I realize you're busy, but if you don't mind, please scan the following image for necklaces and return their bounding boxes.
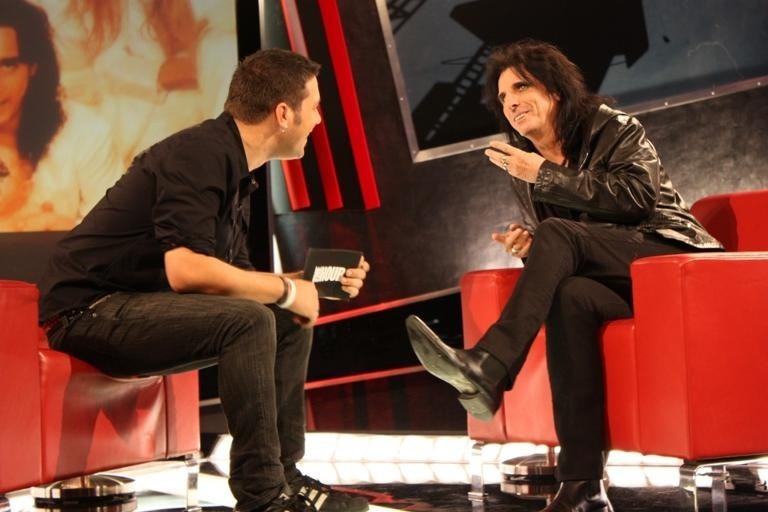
[559,153,568,167]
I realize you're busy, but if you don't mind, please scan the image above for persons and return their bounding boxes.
[0,0,115,234]
[404,36,728,511]
[33,45,372,512]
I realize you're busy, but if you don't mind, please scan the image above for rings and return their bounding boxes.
[511,246,520,254]
[500,163,508,172]
[501,155,509,164]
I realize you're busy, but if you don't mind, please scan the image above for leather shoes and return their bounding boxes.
[540,480,616,512]
[404,313,509,423]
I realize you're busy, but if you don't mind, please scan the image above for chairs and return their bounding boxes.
[1,278,202,510]
[458,188,767,504]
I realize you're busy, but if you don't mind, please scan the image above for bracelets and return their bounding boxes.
[297,269,304,280]
[274,276,297,310]
[276,275,289,306]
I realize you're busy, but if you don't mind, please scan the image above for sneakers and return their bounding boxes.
[291,474,369,512]
[232,486,316,512]
